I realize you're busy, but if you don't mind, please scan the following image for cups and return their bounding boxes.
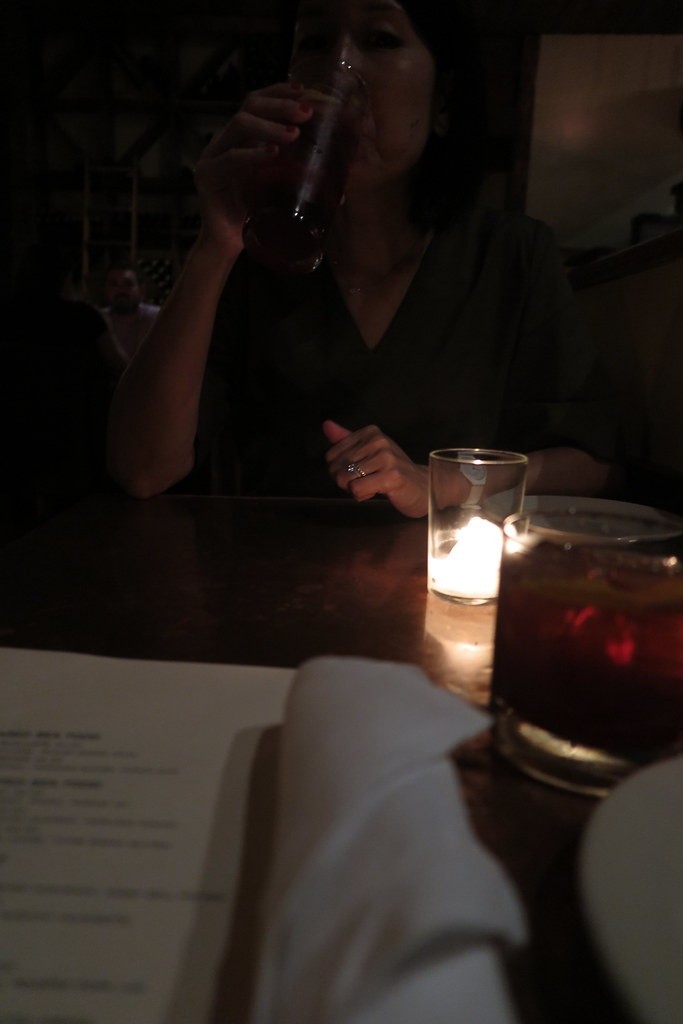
[426,447,528,603]
[243,55,373,271]
[485,507,683,798]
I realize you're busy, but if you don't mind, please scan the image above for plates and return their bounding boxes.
[523,494,683,549]
[576,756,683,1023]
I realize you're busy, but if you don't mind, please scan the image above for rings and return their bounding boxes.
[345,463,366,477]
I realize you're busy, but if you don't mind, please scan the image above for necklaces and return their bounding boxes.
[347,267,394,294]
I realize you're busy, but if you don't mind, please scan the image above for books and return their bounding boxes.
[0,647,531,1023]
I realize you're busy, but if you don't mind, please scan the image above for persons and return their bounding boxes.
[0,243,162,549]
[106,1,649,520]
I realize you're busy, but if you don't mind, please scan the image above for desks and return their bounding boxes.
[0,493,426,668]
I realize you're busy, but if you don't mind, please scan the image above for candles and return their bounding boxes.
[426,448,529,605]
